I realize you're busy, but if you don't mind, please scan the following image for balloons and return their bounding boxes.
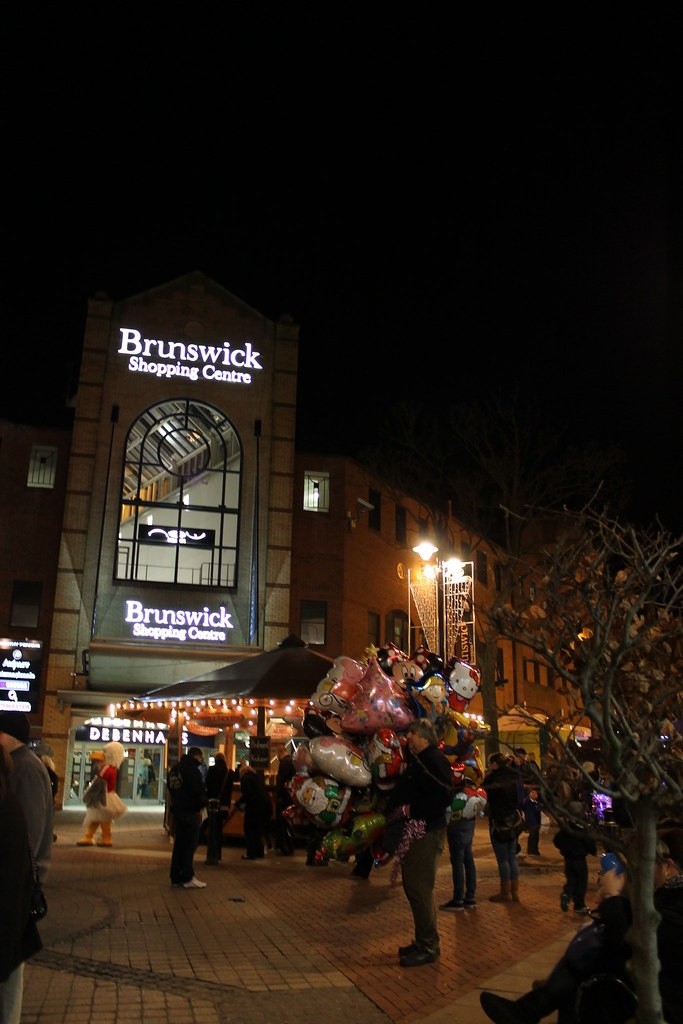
[280,640,489,846]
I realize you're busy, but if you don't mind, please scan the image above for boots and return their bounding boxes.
[489,883,512,902]
[512,882,520,901]
[480,992,538,1024]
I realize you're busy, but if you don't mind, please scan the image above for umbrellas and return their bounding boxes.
[130,629,335,703]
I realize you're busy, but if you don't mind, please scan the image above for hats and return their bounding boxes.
[0,710,29,744]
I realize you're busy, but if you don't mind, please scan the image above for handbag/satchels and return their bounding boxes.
[208,799,220,812]
[29,883,49,922]
[505,809,526,833]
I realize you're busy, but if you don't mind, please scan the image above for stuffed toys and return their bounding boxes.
[74,741,129,847]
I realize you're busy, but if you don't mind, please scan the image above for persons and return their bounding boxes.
[166,748,207,889]
[479,850,683,1023]
[0,710,59,1024]
[235,765,274,859]
[440,764,478,909]
[390,721,453,964]
[552,802,603,915]
[137,757,157,799]
[268,746,295,856]
[204,753,241,864]
[304,822,375,881]
[483,745,543,903]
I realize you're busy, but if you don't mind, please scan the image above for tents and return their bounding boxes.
[479,703,573,769]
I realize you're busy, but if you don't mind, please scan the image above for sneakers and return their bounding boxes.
[439,900,464,911]
[463,899,477,908]
[399,943,440,967]
[171,877,207,888]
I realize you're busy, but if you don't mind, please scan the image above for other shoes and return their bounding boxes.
[242,854,250,859]
[561,893,568,911]
[528,851,540,855]
[575,907,587,912]
[204,856,221,865]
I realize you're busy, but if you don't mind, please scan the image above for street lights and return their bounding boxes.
[412,514,466,667]
